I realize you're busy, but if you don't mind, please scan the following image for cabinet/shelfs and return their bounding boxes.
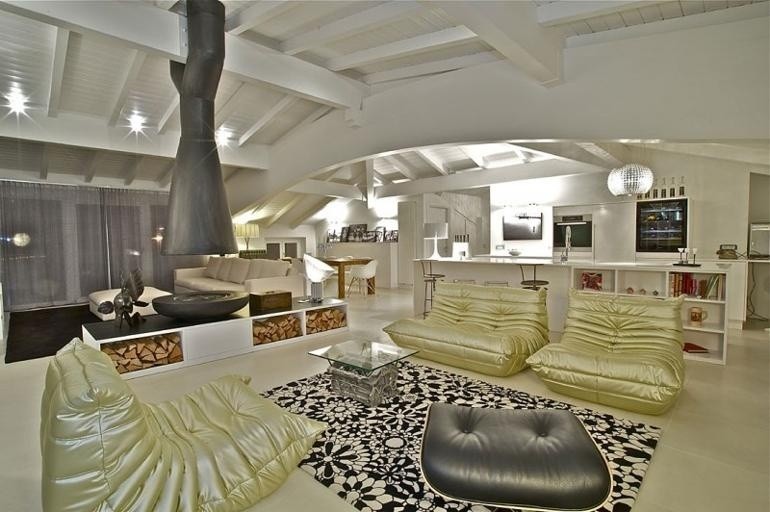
[567,267,729,367]
[82,301,350,380]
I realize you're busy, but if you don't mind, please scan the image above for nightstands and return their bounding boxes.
[308,339,420,408]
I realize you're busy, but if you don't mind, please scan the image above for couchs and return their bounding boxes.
[171,263,303,297]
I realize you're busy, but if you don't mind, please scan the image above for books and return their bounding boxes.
[682,342,709,353]
[670,272,725,300]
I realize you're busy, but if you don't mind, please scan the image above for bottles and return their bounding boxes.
[637,177,685,199]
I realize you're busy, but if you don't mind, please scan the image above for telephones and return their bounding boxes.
[717,249,737,259]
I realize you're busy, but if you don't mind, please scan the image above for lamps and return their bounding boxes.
[603,164,656,199]
[302,252,334,303]
[233,221,260,249]
[423,220,450,260]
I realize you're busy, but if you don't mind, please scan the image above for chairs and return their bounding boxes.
[319,253,379,297]
[518,263,551,306]
[419,257,443,318]
[37,330,325,510]
[532,288,686,415]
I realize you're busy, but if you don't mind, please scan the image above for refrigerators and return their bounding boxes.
[634,200,687,257]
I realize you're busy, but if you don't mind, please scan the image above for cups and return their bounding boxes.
[690,306,709,328]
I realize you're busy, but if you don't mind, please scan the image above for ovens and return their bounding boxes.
[553,215,594,253]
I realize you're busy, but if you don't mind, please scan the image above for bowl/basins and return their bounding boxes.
[152,289,250,319]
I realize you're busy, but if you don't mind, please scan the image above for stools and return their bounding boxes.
[418,403,613,511]
[88,286,173,321]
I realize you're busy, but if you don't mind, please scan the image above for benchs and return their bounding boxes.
[381,278,549,378]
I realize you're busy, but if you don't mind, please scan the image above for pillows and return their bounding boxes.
[203,256,286,282]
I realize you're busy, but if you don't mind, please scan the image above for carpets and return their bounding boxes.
[259,362,665,512]
[2,303,102,364]
